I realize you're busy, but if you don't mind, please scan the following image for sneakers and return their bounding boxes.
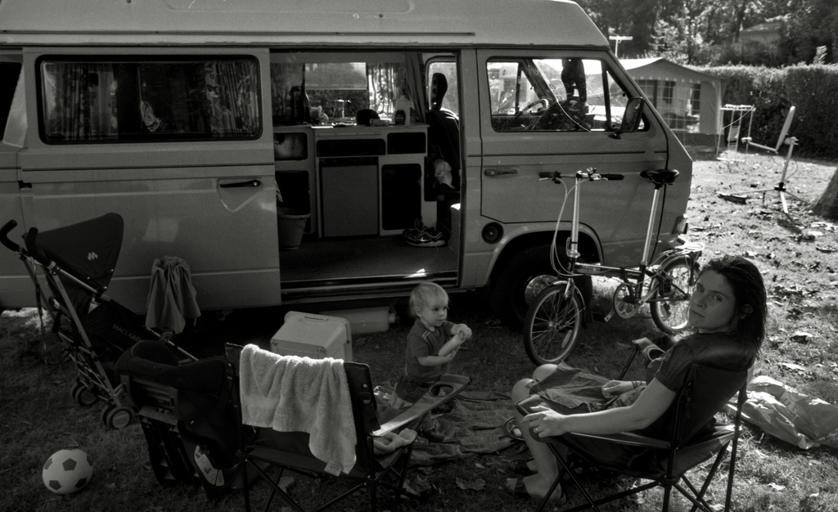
[421,427,444,441]
[406,226,446,247]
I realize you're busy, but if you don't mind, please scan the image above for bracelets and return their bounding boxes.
[632,380,643,389]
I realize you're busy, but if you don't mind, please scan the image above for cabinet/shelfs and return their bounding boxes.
[273,126,321,240]
[377,123,432,235]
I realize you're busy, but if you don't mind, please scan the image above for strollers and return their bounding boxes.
[1,204,197,431]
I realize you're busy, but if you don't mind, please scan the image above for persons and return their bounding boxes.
[283,86,329,124]
[503,254,768,506]
[396,282,477,415]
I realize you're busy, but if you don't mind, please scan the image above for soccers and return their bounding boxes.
[41,450,94,495]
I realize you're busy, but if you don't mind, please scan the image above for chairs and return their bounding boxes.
[428,74,463,185]
[518,318,745,512]
[228,344,472,512]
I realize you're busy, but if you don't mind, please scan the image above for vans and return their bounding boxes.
[1,5,691,336]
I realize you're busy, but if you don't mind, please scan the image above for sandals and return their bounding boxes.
[510,459,538,475]
[504,477,566,505]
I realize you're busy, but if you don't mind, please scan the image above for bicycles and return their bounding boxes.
[520,160,715,370]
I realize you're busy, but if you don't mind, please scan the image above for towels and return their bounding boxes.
[236,347,363,470]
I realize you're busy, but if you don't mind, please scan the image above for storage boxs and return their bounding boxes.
[270,309,353,363]
